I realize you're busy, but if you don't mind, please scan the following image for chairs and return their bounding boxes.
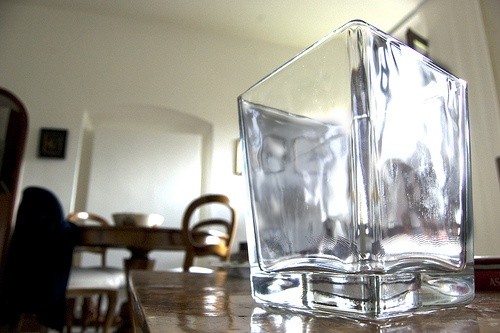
[183,193,237,270]
[68,212,109,332]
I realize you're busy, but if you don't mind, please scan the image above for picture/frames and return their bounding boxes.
[38,128,67,158]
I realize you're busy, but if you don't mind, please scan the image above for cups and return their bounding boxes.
[236,19,475,322]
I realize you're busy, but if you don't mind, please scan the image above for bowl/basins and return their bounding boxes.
[111,212,165,228]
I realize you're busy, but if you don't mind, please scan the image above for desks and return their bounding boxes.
[128,269,500,333]
[71,226,186,333]
